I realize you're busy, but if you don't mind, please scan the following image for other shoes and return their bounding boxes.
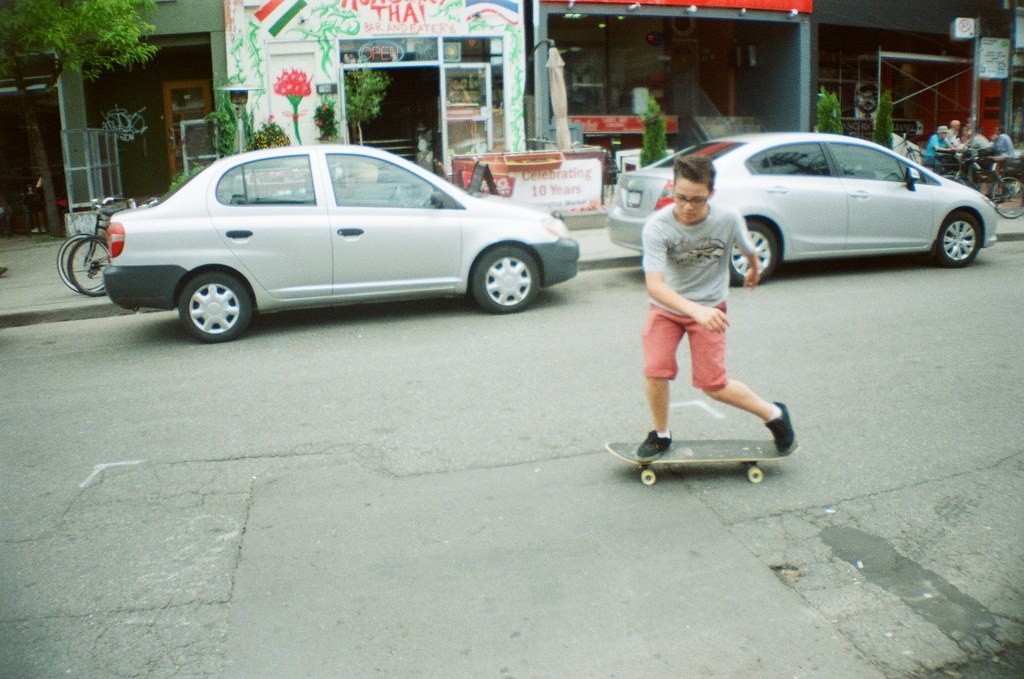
[0,265,7,276]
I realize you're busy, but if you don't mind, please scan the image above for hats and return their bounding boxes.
[937,126,950,133]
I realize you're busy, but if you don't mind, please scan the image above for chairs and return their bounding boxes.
[0,186,49,238]
[934,150,1024,183]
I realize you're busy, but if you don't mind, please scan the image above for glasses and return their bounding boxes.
[673,194,710,208]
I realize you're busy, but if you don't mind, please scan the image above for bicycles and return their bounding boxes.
[56,197,155,297]
[941,151,1024,219]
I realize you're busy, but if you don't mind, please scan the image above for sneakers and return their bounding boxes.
[636,430,672,462]
[766,402,796,456]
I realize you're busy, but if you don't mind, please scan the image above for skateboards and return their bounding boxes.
[604,438,801,487]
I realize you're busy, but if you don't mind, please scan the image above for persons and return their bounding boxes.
[635,156,796,463]
[922,120,1014,174]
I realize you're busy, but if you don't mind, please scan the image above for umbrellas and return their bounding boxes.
[546,39,572,150]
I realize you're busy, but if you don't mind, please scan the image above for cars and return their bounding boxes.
[604,131,997,286]
[101,143,581,345]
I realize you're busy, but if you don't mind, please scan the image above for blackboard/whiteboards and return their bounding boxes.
[468,161,498,195]
